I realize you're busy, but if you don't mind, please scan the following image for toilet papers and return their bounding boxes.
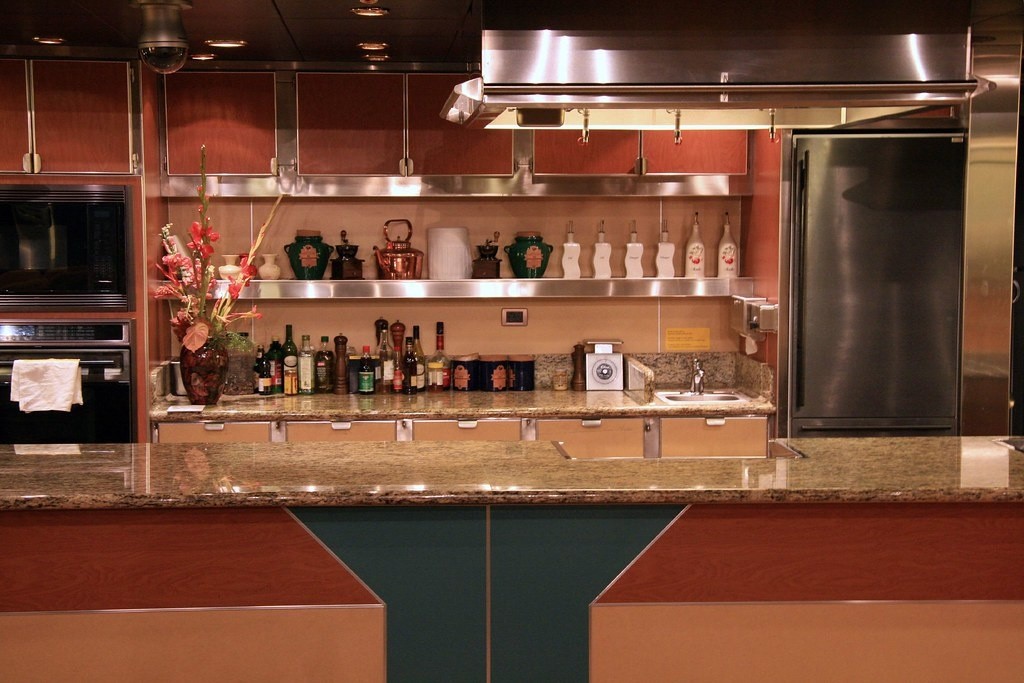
[427,226,475,280]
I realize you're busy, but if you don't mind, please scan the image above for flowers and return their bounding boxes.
[152,144,283,354]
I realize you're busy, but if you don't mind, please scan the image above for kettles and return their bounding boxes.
[373,218,423,280]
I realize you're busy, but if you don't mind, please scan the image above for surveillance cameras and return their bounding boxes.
[137,3,190,74]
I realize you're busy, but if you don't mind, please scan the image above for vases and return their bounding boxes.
[180,339,228,405]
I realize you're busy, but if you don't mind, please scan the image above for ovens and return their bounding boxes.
[1,318,133,445]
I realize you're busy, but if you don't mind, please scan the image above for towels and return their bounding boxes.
[10,358,84,414]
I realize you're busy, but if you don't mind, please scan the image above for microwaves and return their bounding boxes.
[1,183,134,312]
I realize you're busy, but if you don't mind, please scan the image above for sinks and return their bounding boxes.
[664,395,740,402]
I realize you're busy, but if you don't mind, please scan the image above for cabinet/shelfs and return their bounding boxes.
[0,57,142,183]
[155,60,754,199]
[159,415,770,458]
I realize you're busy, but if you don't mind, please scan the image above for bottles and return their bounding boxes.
[504,231,553,278]
[684,213,705,278]
[258,253,281,280]
[552,368,568,391]
[717,215,738,278]
[624,220,644,278]
[253,322,451,395]
[238,253,257,279]
[218,254,240,280]
[591,220,612,279]
[655,220,675,278]
[561,220,581,279]
[284,230,334,280]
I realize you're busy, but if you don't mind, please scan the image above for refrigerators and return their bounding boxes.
[782,132,970,438]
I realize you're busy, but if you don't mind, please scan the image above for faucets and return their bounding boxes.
[690,358,706,392]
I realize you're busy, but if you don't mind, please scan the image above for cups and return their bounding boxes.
[451,360,535,391]
